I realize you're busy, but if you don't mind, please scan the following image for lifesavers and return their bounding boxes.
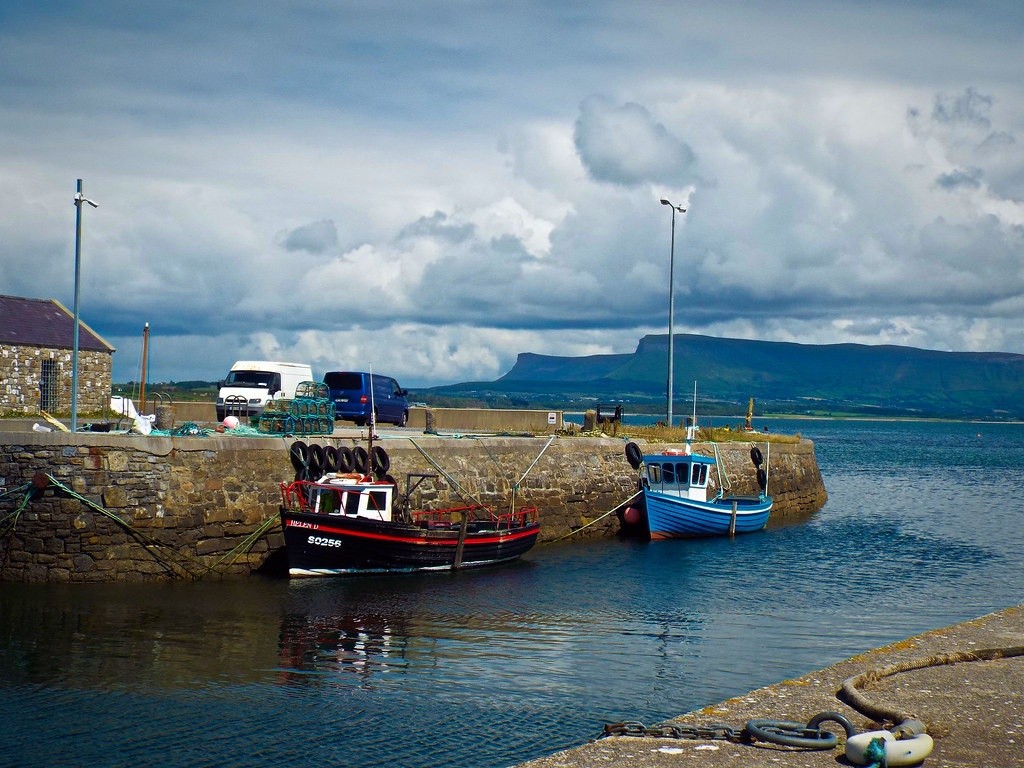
[750,447,764,466]
[625,441,644,466]
[757,468,767,490]
[289,439,399,506]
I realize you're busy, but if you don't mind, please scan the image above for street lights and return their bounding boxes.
[70,178,101,433]
[659,196,687,427]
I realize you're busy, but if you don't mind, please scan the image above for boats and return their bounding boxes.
[635,379,774,542]
[277,362,543,578]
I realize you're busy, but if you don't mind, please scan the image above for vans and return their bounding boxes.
[322,371,410,427]
[214,360,314,422]
